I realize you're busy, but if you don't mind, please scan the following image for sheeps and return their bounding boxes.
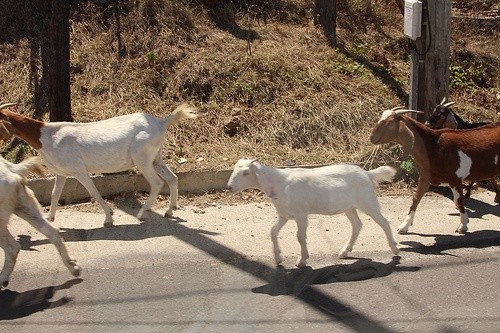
[370,106,500,234]
[0,102,201,227]
[0,154,83,288]
[424,97,500,206]
[227,158,400,268]
